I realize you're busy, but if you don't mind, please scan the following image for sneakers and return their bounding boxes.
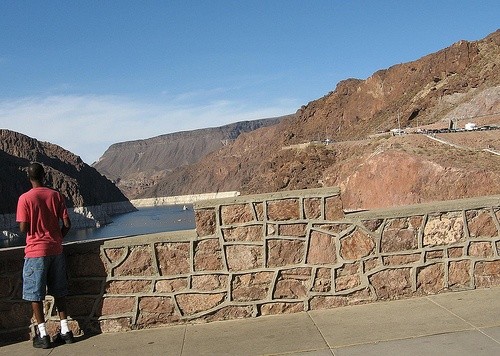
[33,331,51,349]
[52,329,75,344]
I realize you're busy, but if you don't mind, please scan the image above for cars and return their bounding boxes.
[427,123,495,134]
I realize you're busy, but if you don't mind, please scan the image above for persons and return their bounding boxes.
[16,162,75,349]
